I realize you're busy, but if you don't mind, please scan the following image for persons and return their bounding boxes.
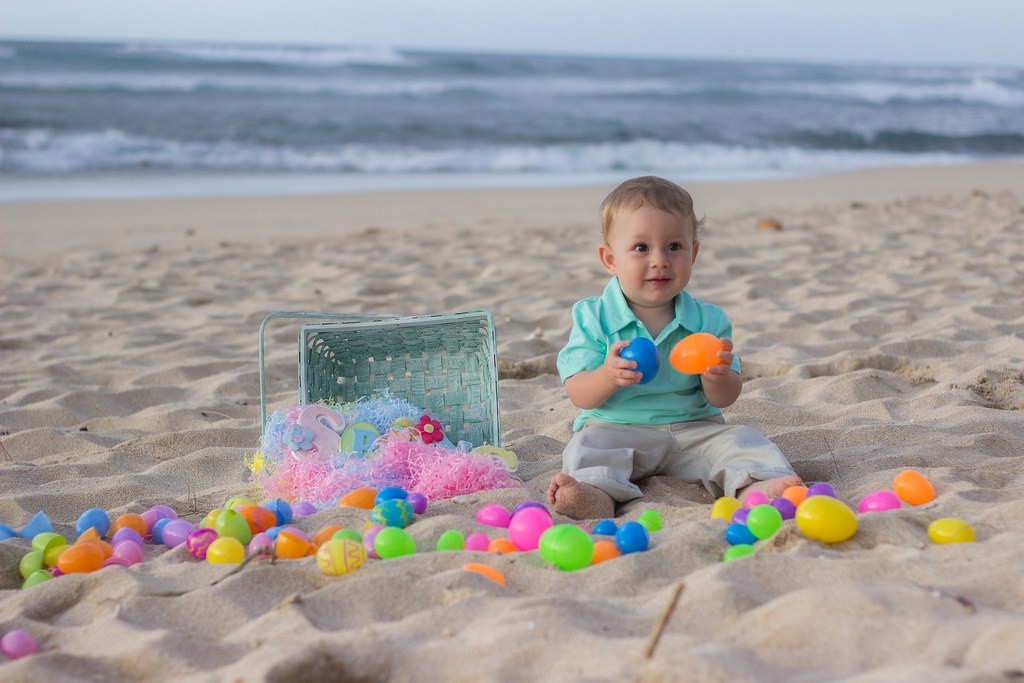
[547,175,806,521]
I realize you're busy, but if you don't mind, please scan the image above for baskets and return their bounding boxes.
[258,311,500,454]
